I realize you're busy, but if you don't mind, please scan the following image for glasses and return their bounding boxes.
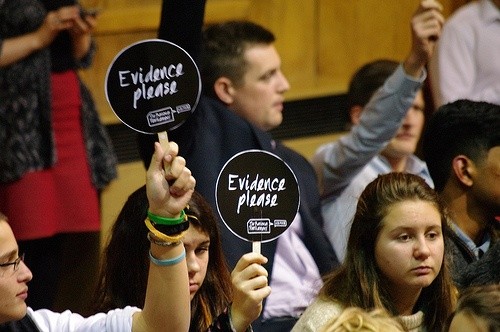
[0,253,24,271]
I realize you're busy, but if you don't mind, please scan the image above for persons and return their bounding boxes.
[291,0,500,332]
[90,180,271,332]
[0,0,117,318]
[135,1,341,332]
[0,141,196,332]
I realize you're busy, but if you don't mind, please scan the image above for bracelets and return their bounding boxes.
[145,208,189,266]
